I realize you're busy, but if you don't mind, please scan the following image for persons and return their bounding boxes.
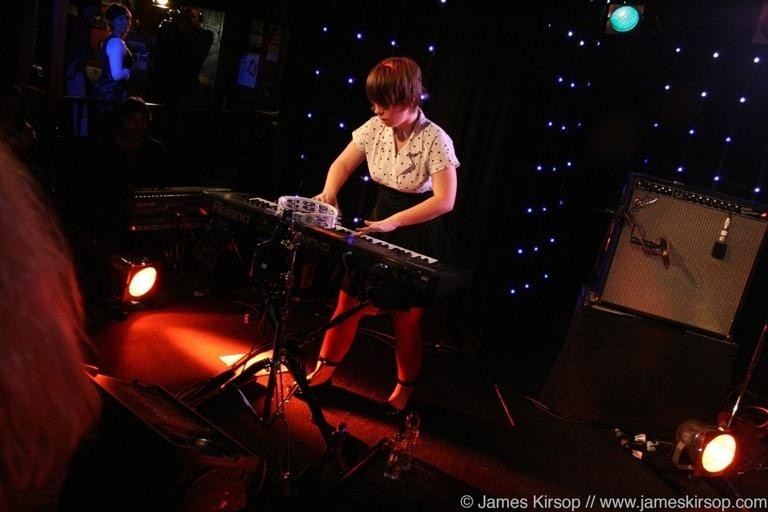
[0,85,36,158]
[287,56,461,421]
[63,0,214,104]
[0,141,103,512]
[104,96,167,186]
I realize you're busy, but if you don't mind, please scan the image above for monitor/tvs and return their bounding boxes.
[235,47,262,91]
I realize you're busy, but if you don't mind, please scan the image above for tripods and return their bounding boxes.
[194,251,337,449]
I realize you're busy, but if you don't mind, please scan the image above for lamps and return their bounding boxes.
[669,416,738,477]
[99,249,160,305]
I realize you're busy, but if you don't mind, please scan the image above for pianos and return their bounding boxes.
[212,192,450,295]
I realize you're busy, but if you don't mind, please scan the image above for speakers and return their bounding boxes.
[597,168,768,340]
[80,370,260,512]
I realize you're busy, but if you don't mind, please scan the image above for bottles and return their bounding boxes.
[615,428,632,456]
[384,409,420,481]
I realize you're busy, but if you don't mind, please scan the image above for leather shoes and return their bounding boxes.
[376,388,415,418]
[287,376,333,396]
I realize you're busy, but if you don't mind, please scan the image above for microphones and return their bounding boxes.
[710,212,734,258]
[630,235,670,258]
